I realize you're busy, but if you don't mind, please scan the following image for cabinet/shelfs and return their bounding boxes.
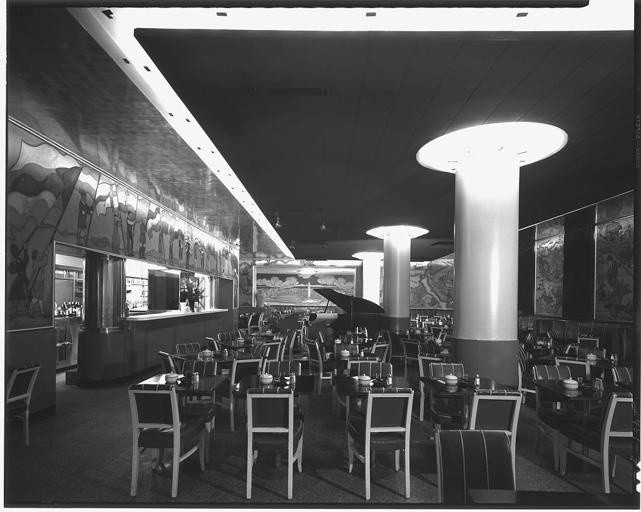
[54,317,82,370]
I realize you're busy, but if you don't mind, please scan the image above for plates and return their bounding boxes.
[284,376,290,380]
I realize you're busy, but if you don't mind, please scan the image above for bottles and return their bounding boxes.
[250,338,253,345]
[224,349,228,357]
[385,376,389,386]
[290,373,296,384]
[57,305,63,316]
[55,300,58,316]
[474,374,481,387]
[405,329,409,334]
[191,373,197,383]
[195,372,200,382]
[595,378,599,390]
[598,379,603,391]
[198,352,203,360]
[274,333,277,340]
[63,300,81,317]
[387,374,392,385]
[278,332,281,339]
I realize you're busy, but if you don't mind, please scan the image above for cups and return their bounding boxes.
[360,349,365,358]
[256,368,263,377]
[487,379,495,392]
[350,337,354,345]
[352,377,359,385]
[211,351,215,358]
[193,307,201,312]
[194,302,198,307]
[577,376,583,382]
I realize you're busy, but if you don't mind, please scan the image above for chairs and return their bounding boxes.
[5,363,41,447]
